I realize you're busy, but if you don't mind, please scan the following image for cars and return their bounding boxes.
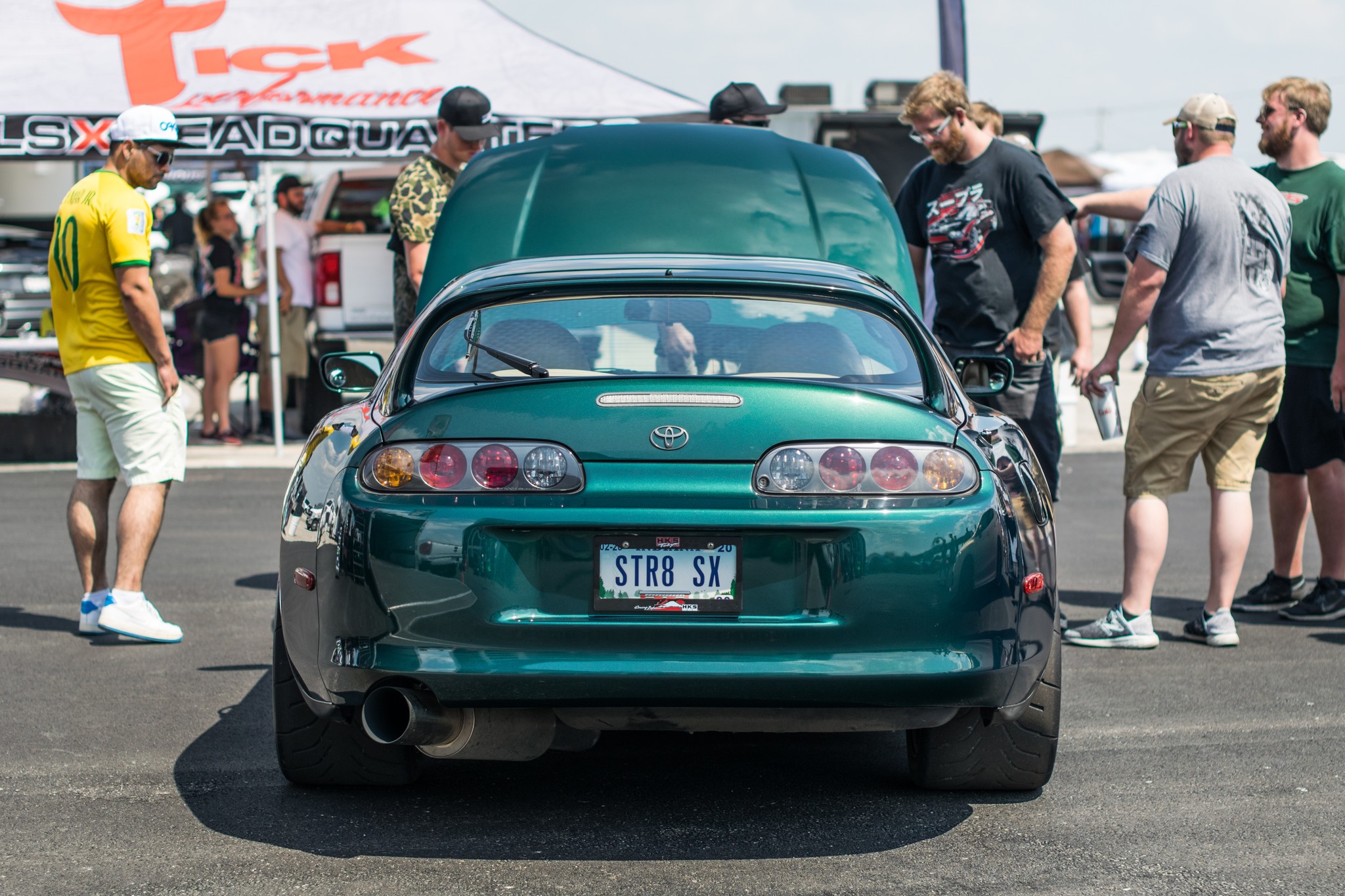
[271,121,1065,806]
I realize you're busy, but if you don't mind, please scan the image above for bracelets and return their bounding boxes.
[344,222,354,232]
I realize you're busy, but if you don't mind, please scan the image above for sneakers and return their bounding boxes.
[1183,605,1240,647]
[78,598,108,634]
[1277,574,1345,621]
[97,592,184,643]
[1063,603,1160,650]
[1231,568,1309,612]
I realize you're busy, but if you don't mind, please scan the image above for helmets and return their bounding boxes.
[110,104,180,143]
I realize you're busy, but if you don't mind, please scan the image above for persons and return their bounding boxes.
[654,323,698,373]
[386,86,501,347]
[1064,93,1292,648]
[1070,76,1345,621]
[709,82,788,128]
[892,69,1094,630]
[161,174,365,445]
[47,105,187,643]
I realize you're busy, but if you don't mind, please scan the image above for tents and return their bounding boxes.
[0,0,709,454]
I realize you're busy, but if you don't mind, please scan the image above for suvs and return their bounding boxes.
[292,162,411,342]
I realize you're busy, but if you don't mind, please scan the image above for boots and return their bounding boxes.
[253,408,275,445]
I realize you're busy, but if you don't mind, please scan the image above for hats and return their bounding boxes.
[709,81,789,122]
[276,175,312,195]
[437,85,501,141]
[1163,92,1237,134]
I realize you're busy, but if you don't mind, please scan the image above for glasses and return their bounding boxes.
[1260,104,1298,122]
[1172,120,1194,138]
[134,140,174,166]
[909,109,967,144]
[728,119,771,128]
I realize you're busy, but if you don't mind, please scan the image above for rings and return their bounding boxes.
[1087,381,1093,386]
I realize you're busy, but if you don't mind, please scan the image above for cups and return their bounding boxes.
[1089,380,1123,440]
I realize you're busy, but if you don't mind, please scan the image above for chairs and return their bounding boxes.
[737,322,867,377]
[463,319,594,372]
[167,297,260,437]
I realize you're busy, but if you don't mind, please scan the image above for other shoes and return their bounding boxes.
[199,426,242,446]
